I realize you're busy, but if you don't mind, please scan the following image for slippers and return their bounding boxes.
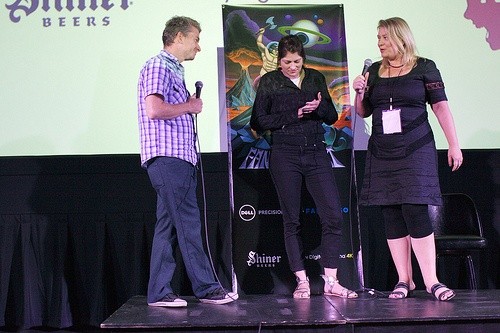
[387,281,413,298]
[431,282,456,301]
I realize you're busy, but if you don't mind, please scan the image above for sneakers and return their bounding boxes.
[199,286,239,304]
[148,293,188,307]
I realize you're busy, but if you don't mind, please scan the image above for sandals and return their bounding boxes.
[293,276,310,299]
[320,274,358,299]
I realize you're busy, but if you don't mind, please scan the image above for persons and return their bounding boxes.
[138,15,239,307]
[353,17,464,301]
[250,34,358,299]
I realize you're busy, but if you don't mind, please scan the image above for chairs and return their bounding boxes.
[436,192,488,290]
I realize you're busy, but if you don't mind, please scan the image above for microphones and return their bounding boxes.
[195,81,203,115]
[358,59,372,94]
